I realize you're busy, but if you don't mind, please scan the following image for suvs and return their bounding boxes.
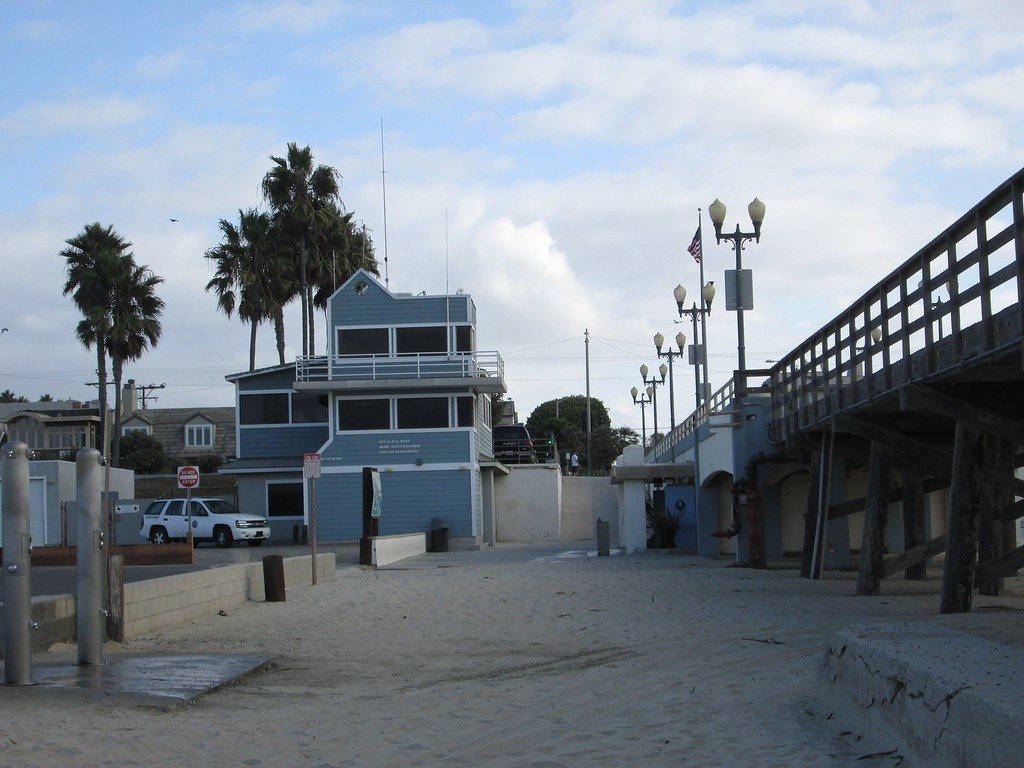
[492,423,536,464]
[139,495,272,549]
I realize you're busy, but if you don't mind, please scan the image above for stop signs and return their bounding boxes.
[176,466,199,489]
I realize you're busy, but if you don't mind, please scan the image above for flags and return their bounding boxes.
[687,227,700,263]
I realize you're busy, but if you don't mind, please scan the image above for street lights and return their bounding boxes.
[706,196,768,395]
[629,386,654,453]
[639,363,668,445]
[918,277,959,339]
[652,332,687,431]
[853,325,883,376]
[673,280,717,410]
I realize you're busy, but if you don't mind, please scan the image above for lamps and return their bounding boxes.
[356,282,369,296]
[417,291,426,296]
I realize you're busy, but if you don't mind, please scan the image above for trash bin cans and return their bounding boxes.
[431,516,448,552]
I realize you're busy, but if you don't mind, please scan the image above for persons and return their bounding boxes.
[571,451,580,477]
